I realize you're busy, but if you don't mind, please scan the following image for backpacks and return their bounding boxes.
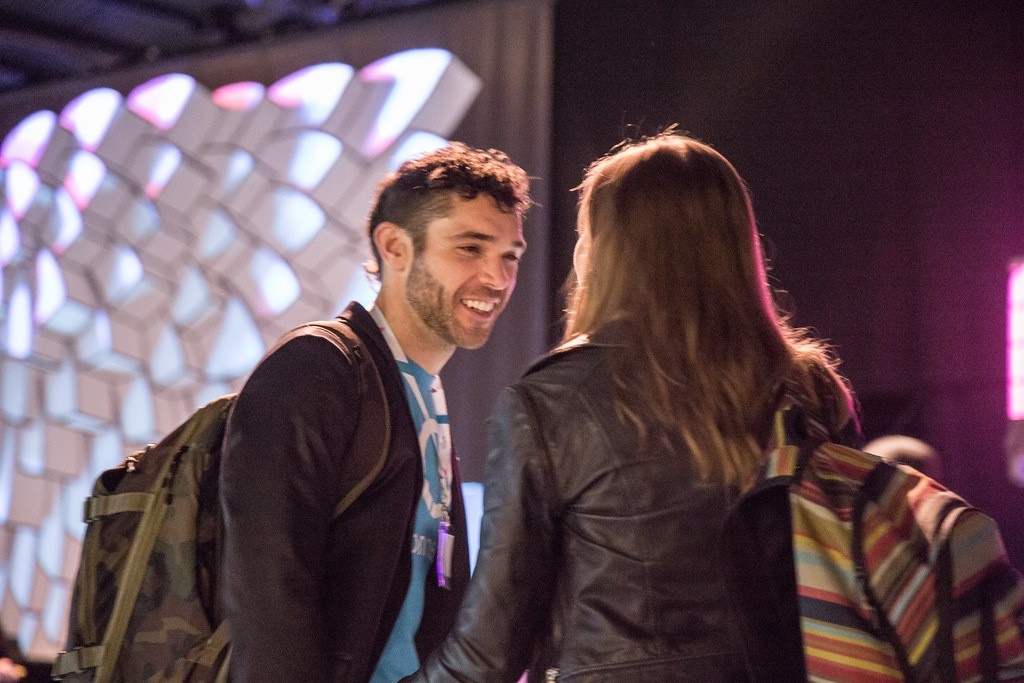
[716,387,1024,683]
[50,318,392,683]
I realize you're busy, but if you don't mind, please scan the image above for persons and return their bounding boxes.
[218,143,537,683]
[384,130,866,683]
